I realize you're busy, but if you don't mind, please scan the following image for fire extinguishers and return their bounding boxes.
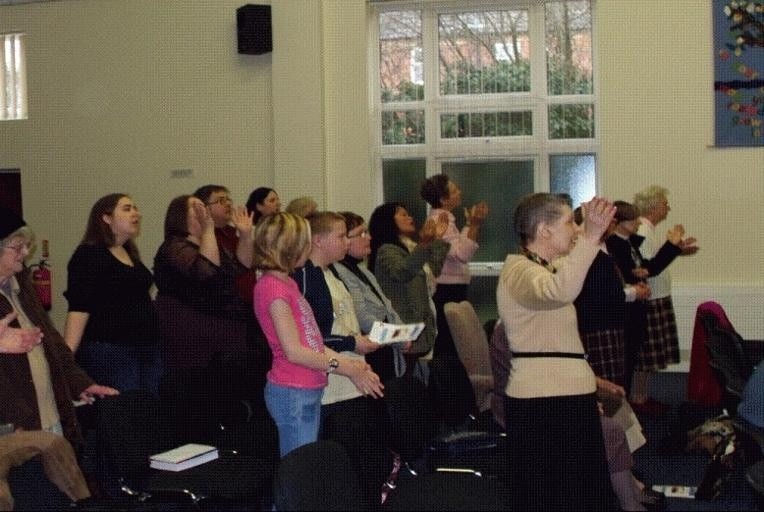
[28,258,51,311]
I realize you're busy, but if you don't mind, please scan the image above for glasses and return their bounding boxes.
[206,196,226,207]
[3,242,25,252]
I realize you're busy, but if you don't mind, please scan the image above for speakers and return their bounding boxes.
[236,4,272,55]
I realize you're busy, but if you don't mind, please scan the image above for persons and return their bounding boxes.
[737,358,764,491]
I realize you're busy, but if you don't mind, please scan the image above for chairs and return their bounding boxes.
[695,301,764,512]
[60,348,280,512]
[273,301,508,512]
